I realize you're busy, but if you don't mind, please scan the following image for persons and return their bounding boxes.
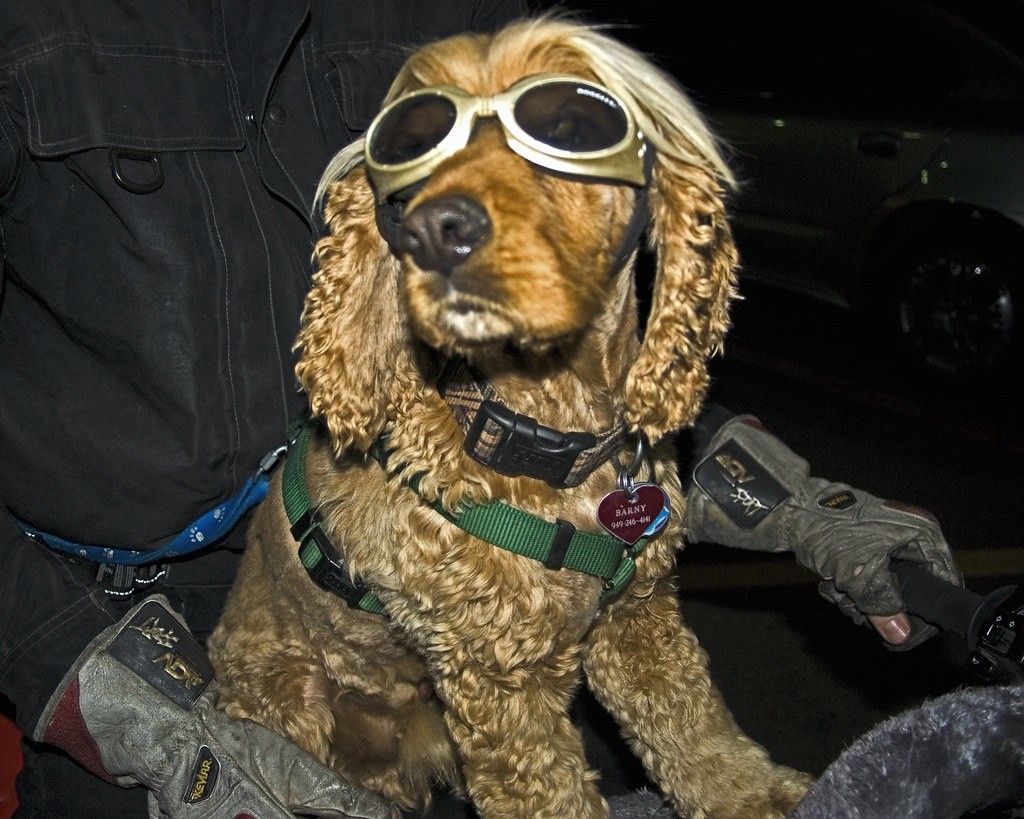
[0,0,965,819]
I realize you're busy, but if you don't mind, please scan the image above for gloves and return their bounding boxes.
[28,594,392,819]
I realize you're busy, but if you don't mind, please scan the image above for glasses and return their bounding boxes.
[362,75,653,208]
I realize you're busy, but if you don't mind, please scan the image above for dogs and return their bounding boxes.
[206,13,818,819]
